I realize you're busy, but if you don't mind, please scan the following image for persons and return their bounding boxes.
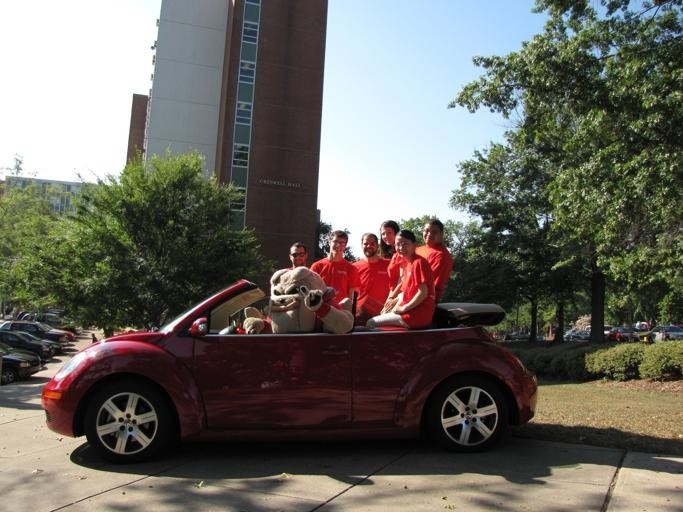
[365,229,434,329]
[307,231,360,312]
[380,220,400,262]
[414,219,455,303]
[351,233,390,322]
[287,241,310,270]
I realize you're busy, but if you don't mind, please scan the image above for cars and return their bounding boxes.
[567,322,683,350]
[494,330,529,342]
[0,306,79,383]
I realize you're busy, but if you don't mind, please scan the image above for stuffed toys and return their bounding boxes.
[242,266,354,335]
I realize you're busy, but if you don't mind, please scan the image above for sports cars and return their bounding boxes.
[41,274,538,456]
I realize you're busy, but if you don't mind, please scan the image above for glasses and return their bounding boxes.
[291,252,305,257]
